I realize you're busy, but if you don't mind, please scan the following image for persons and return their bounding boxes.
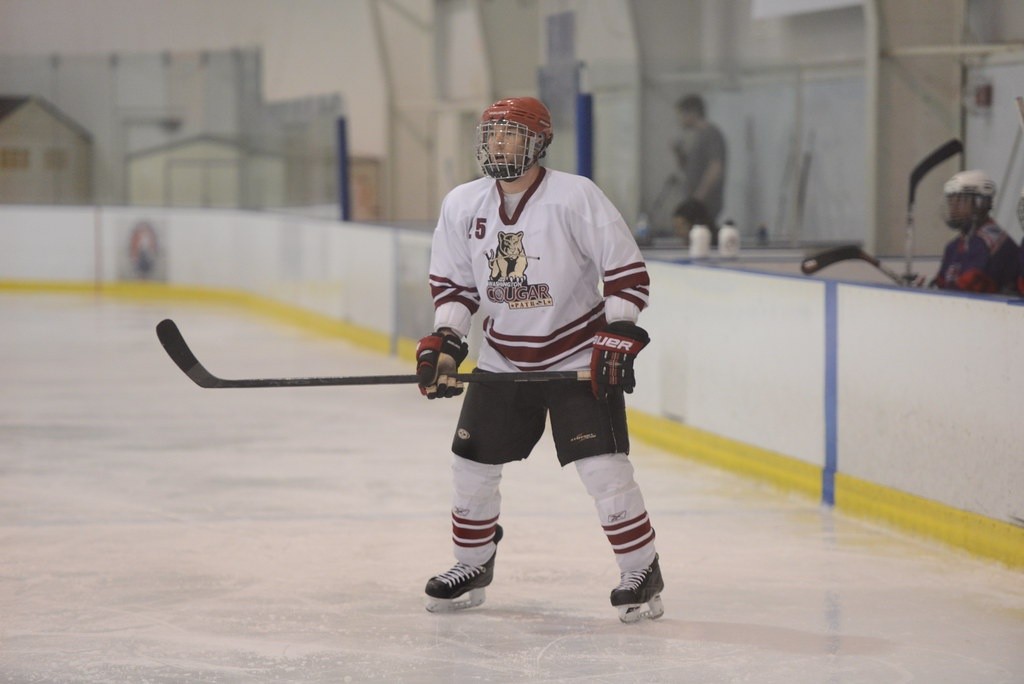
[415,97,665,622]
[902,168,1019,296]
[670,94,727,243]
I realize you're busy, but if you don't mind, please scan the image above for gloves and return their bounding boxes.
[415,331,469,401]
[590,322,651,405]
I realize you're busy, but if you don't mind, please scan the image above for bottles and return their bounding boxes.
[690,218,712,256]
[717,219,740,254]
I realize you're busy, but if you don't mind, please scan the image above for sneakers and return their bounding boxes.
[612,553,666,625]
[424,523,503,613]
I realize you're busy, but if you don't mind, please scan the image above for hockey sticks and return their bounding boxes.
[155,317,592,389]
[801,244,908,286]
[906,137,963,284]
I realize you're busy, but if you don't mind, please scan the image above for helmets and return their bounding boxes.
[938,171,998,231]
[474,97,554,184]
